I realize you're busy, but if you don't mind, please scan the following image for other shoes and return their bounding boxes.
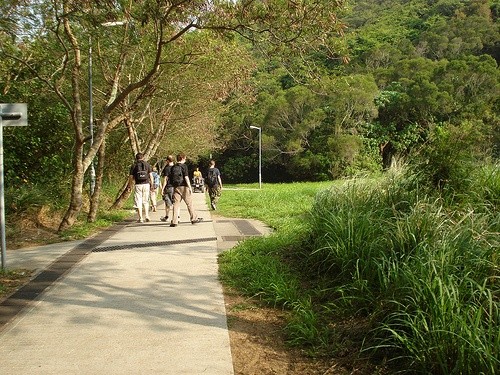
[211,203,217,210]
[136,218,143,223]
[152,205,156,212]
[160,216,166,221]
[145,216,149,222]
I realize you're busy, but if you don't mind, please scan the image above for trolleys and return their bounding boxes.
[192,176,205,193]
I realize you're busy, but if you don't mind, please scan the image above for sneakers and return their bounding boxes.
[191,218,204,225]
[170,221,179,227]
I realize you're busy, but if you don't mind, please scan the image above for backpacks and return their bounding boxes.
[135,160,148,180]
[205,170,217,185]
[170,164,183,187]
[152,173,159,189]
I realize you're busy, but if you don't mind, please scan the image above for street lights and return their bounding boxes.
[250,125,262,189]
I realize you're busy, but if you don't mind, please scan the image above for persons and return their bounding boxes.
[149,167,162,211]
[127,153,153,223]
[159,155,181,223]
[170,153,203,227]
[193,168,202,178]
[206,161,222,210]
